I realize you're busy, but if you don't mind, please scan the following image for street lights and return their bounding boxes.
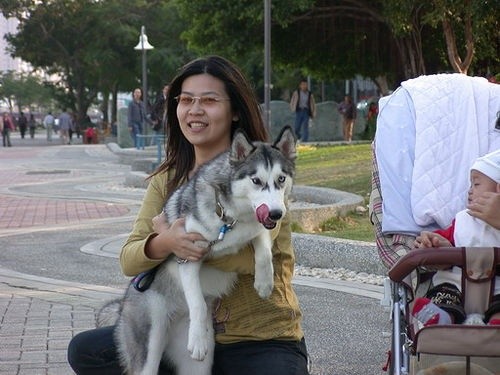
[132,25,155,135]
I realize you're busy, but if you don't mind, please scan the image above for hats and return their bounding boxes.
[469,150,500,183]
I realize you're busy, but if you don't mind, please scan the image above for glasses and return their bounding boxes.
[175,94,234,107]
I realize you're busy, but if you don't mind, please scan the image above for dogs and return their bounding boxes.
[87,121,301,374]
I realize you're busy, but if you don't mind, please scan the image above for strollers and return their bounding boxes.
[366,71,500,375]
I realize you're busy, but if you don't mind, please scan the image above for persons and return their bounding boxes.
[412,151,500,327]
[59,108,73,144]
[45,112,55,141]
[369,103,378,117]
[291,80,316,142]
[151,84,169,131]
[87,125,94,144]
[18,112,36,138]
[338,96,356,141]
[67,56,309,375]
[127,88,153,145]
[0,112,15,146]
